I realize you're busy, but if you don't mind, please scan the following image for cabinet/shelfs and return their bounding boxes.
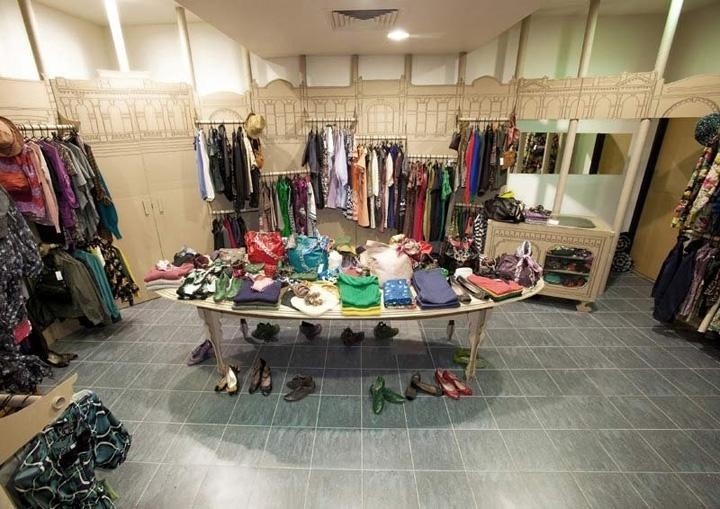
[480,212,616,313]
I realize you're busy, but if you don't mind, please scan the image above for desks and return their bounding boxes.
[153,253,546,383]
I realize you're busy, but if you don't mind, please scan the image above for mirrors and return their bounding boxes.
[510,131,633,176]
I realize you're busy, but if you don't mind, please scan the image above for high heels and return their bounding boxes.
[186,340,214,365]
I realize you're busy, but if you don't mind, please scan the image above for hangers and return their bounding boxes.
[308,116,358,136]
[254,167,311,183]
[454,202,478,216]
[195,116,246,137]
[353,136,403,150]
[407,152,457,167]
[458,116,504,129]
[16,121,79,151]
[211,207,260,219]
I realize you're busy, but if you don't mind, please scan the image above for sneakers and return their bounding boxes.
[251,323,280,339]
[372,322,398,339]
[451,346,488,369]
[340,329,364,346]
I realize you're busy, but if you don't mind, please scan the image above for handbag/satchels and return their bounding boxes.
[484,191,526,224]
[37,282,72,308]
[436,238,479,273]
[498,149,516,170]
[244,231,330,276]
[495,241,544,287]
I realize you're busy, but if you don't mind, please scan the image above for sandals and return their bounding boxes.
[448,275,471,305]
[457,275,489,301]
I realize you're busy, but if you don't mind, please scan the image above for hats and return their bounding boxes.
[0,116,24,158]
[245,113,266,140]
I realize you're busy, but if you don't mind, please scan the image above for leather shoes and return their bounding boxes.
[227,276,241,300]
[406,370,473,402]
[46,349,77,367]
[213,271,229,302]
[214,365,240,396]
[283,376,315,401]
[371,369,403,414]
[248,358,273,395]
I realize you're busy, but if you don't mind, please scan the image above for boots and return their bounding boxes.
[298,322,321,341]
